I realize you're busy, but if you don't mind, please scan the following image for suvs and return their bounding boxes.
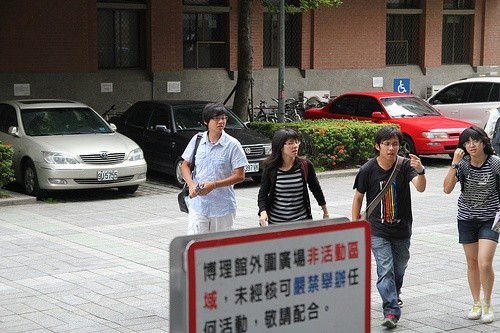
[421,72,500,156]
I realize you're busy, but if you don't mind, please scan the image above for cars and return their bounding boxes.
[108,99,273,189]
[0,98,148,195]
[303,91,475,160]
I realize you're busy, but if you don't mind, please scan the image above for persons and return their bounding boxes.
[444,126,500,323]
[179,103,251,236]
[353,127,427,329]
[257,128,330,227]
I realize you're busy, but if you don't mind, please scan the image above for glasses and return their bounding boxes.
[463,140,483,146]
[381,143,400,148]
[284,141,301,145]
[211,116,228,121]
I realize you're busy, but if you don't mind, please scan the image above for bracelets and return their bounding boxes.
[214,181,216,188]
[322,207,327,210]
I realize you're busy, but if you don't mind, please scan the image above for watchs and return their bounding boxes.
[451,162,459,169]
[417,166,426,175]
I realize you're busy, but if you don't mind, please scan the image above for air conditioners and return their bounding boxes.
[297,90,330,110]
[425,85,446,99]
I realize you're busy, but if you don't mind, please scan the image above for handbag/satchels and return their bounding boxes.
[178,186,190,214]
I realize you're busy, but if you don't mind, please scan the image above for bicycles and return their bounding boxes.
[226,94,338,129]
[101,104,126,121]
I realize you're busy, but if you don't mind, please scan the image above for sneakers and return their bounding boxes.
[467,305,481,320]
[481,305,495,324]
[381,314,398,328]
[397,297,404,308]
